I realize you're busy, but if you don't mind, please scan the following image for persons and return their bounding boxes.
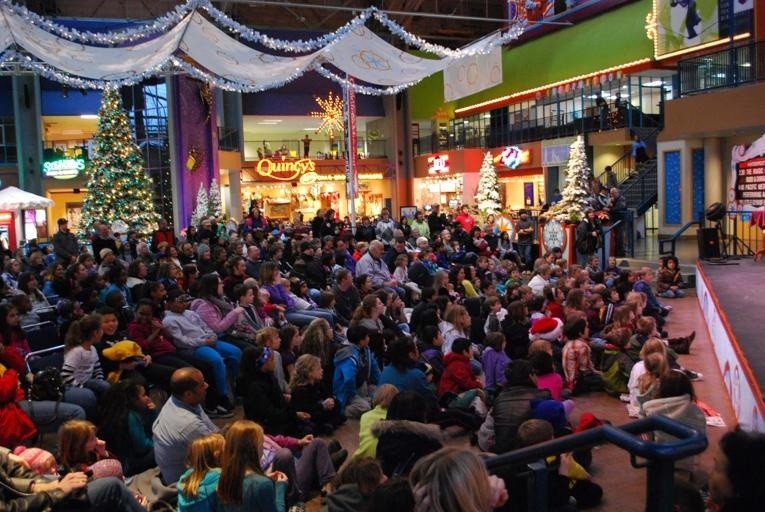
[552,92,651,209]
[257,134,313,159]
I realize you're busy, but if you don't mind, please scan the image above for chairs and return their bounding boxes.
[0,231,145,409]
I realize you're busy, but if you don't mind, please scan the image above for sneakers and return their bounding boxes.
[202,404,235,419]
[321,482,337,496]
[680,365,704,381]
[288,502,306,512]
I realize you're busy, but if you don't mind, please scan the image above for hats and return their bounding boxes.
[299,278,307,285]
[415,236,429,246]
[57,217,68,225]
[98,247,113,260]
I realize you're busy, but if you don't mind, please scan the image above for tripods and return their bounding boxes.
[721,215,755,257]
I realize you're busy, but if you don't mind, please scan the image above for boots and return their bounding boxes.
[668,329,698,355]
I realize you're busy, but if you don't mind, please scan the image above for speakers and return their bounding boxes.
[697,227,720,259]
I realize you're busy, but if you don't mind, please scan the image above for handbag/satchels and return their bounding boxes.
[23,365,66,429]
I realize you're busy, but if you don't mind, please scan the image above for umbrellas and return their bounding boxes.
[0,186,57,212]
